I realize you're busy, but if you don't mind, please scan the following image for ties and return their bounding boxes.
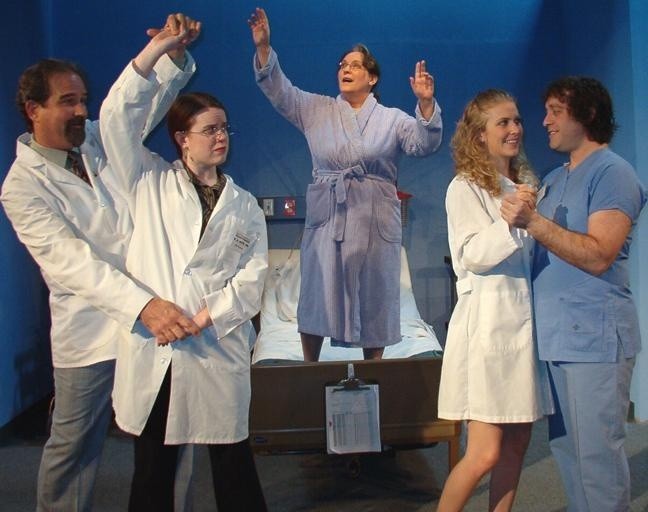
[68,151,88,182]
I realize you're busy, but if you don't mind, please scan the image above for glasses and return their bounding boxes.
[188,123,231,137]
[339,60,362,70]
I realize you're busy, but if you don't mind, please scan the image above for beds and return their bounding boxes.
[250,246,461,473]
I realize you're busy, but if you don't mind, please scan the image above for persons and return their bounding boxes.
[432,87,544,511]
[242,5,447,364]
[496,73,646,511]
[0,8,202,510]
[94,24,274,510]
